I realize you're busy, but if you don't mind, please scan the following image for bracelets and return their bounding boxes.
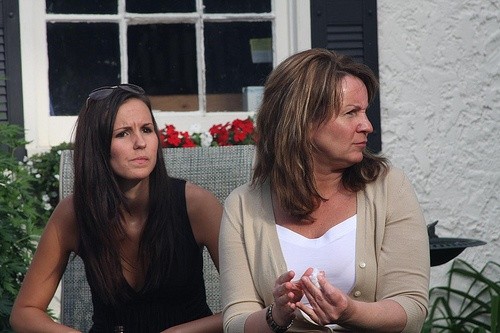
[266,303,294,332]
[334,295,356,326]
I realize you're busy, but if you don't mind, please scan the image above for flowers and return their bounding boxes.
[159,116,258,148]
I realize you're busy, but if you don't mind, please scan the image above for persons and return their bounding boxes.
[9,84,230,333]
[219,47,431,333]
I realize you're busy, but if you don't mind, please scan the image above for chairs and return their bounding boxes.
[59,144,255,333]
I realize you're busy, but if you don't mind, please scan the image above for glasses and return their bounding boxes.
[86,83,146,107]
